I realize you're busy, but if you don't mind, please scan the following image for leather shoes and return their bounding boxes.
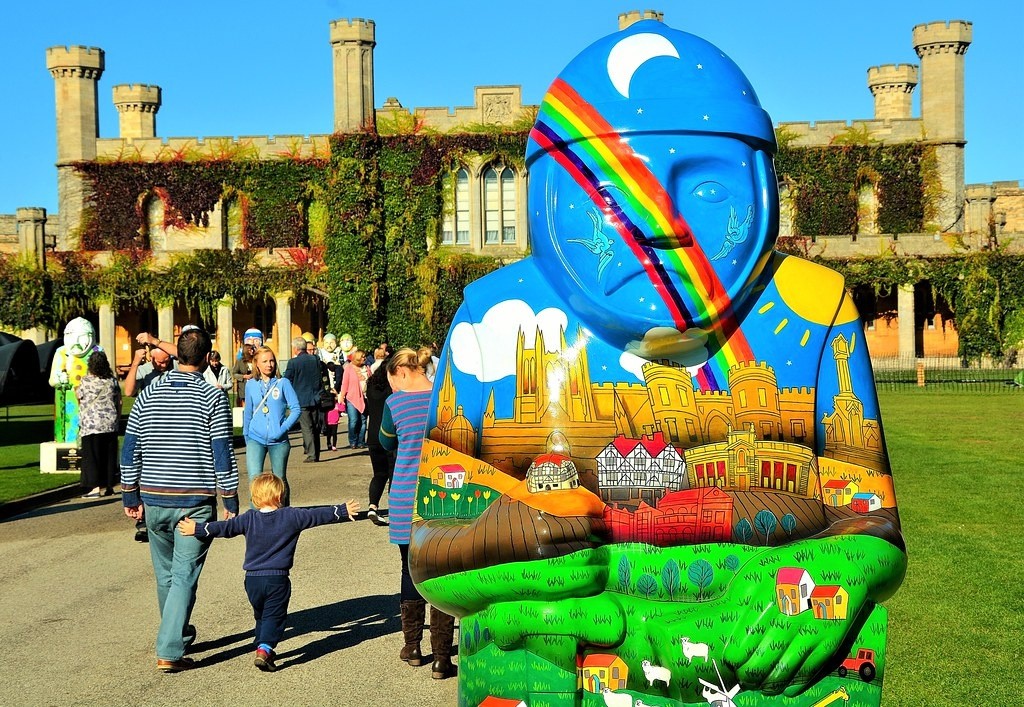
[157,626,196,670]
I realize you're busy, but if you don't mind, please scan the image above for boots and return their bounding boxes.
[399,598,427,666]
[430,604,457,679]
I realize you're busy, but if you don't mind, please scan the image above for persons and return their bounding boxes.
[338,350,366,449]
[178,472,361,672]
[326,361,344,394]
[232,344,254,402]
[306,342,330,436]
[408,19,908,706]
[365,348,401,526]
[283,337,325,463]
[204,350,233,397]
[120,328,240,674]
[243,327,263,349]
[361,342,440,389]
[75,351,123,499]
[49,316,104,443]
[325,388,346,451]
[317,333,343,366]
[339,333,358,363]
[242,345,302,509]
[123,332,181,542]
[380,346,459,679]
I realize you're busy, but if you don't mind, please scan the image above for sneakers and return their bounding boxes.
[367,508,389,525]
[253,647,277,671]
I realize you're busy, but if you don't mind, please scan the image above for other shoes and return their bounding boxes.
[303,458,318,463]
[328,445,337,451]
[135,530,149,542]
[82,493,101,499]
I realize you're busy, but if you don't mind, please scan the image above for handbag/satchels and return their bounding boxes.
[320,392,336,412]
